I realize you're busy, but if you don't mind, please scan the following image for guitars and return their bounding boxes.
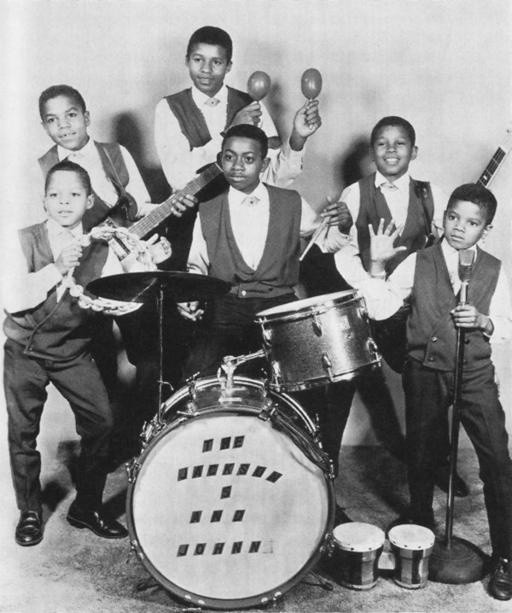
[372,128,512,375]
[88,152,221,278]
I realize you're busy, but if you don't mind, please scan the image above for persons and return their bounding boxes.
[35,83,200,474]
[178,124,353,531]
[153,26,322,271]
[332,115,474,497]
[2,159,156,546]
[366,183,511,602]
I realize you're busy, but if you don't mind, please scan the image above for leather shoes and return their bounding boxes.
[487,553,512,600]
[67,500,128,539]
[15,507,43,546]
[386,516,434,539]
[437,469,468,497]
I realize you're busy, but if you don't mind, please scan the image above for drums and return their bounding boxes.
[256,287,384,391]
[388,523,434,589]
[127,375,337,611]
[330,523,386,591]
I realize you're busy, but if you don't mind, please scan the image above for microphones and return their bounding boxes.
[459,249,475,282]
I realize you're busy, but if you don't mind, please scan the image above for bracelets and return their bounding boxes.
[368,270,387,278]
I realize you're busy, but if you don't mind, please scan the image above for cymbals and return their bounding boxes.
[85,271,231,302]
